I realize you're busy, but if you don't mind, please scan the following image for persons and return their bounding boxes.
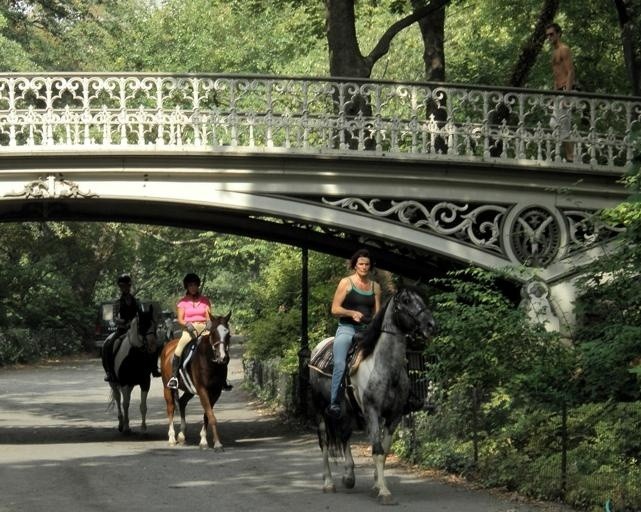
[165,314,175,339]
[167,273,233,391]
[545,23,576,163]
[101,274,161,381]
[329,250,382,412]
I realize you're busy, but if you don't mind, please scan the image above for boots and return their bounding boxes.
[223,385,232,389]
[169,353,182,386]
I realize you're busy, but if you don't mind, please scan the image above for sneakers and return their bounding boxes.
[105,375,115,381]
[153,372,161,376]
[330,404,340,411]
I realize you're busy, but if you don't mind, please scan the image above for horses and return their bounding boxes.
[298,277,436,506]
[160,310,232,453]
[101,304,158,436]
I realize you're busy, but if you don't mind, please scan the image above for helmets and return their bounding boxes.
[118,276,131,286]
[184,273,200,289]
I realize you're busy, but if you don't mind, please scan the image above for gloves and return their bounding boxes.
[187,324,194,332]
[205,320,213,329]
